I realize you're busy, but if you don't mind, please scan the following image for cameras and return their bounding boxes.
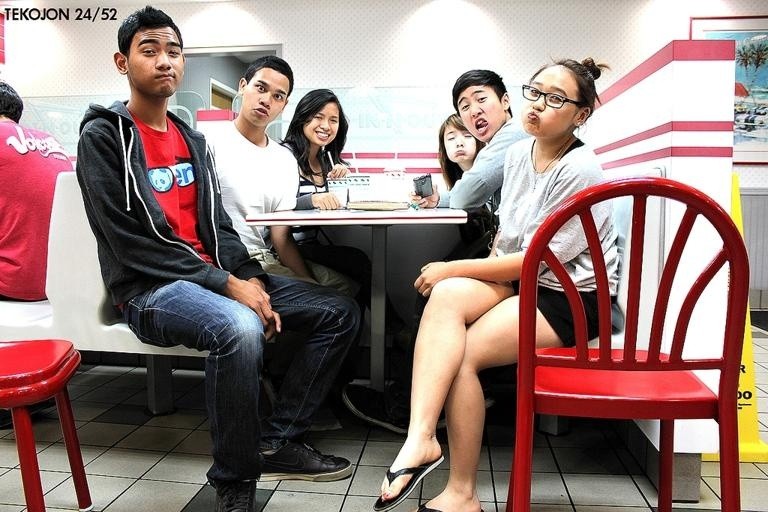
[413,174,433,199]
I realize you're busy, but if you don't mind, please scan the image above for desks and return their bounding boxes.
[243,209,471,444]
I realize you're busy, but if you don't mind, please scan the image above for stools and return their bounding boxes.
[2,339,93,511]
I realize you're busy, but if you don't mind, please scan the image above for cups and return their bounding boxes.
[347,173,372,204]
[382,169,408,202]
[325,176,349,209]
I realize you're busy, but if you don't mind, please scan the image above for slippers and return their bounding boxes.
[416,501,485,512]
[373,453,444,511]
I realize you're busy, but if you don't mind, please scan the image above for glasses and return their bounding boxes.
[522,84,579,110]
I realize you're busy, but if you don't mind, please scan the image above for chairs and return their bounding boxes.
[505,177,752,511]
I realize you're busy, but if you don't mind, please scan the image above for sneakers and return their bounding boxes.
[216,481,256,511]
[341,384,407,434]
[437,379,495,430]
[258,440,352,483]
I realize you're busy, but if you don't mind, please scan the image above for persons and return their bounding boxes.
[370,55,622,511]
[339,68,535,437]
[0,80,74,431]
[416,114,504,321]
[73,5,363,511]
[262,88,406,386]
[200,55,357,432]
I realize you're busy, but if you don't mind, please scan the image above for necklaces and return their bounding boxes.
[311,179,325,185]
[531,134,576,174]
